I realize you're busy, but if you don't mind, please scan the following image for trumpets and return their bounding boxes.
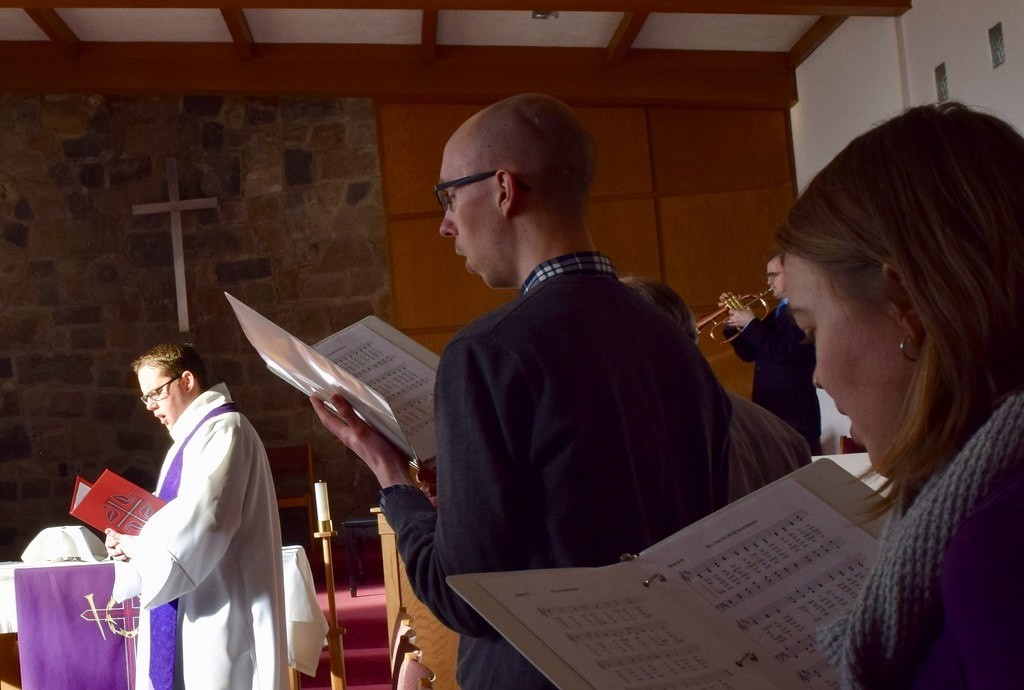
[693,285,775,344]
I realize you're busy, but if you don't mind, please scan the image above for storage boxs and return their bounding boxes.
[377,103,796,402]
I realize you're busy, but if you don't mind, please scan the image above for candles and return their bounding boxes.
[314,480,331,521]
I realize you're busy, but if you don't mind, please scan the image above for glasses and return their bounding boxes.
[141,374,183,404]
[766,270,783,278]
[434,170,532,215]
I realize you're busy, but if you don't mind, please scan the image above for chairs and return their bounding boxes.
[268,442,316,554]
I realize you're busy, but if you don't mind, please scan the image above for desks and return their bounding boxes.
[0,544,306,690]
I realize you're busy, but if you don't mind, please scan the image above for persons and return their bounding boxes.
[721,247,826,456]
[774,103,1023,690]
[619,273,812,507]
[311,91,735,690]
[102,345,289,690]
